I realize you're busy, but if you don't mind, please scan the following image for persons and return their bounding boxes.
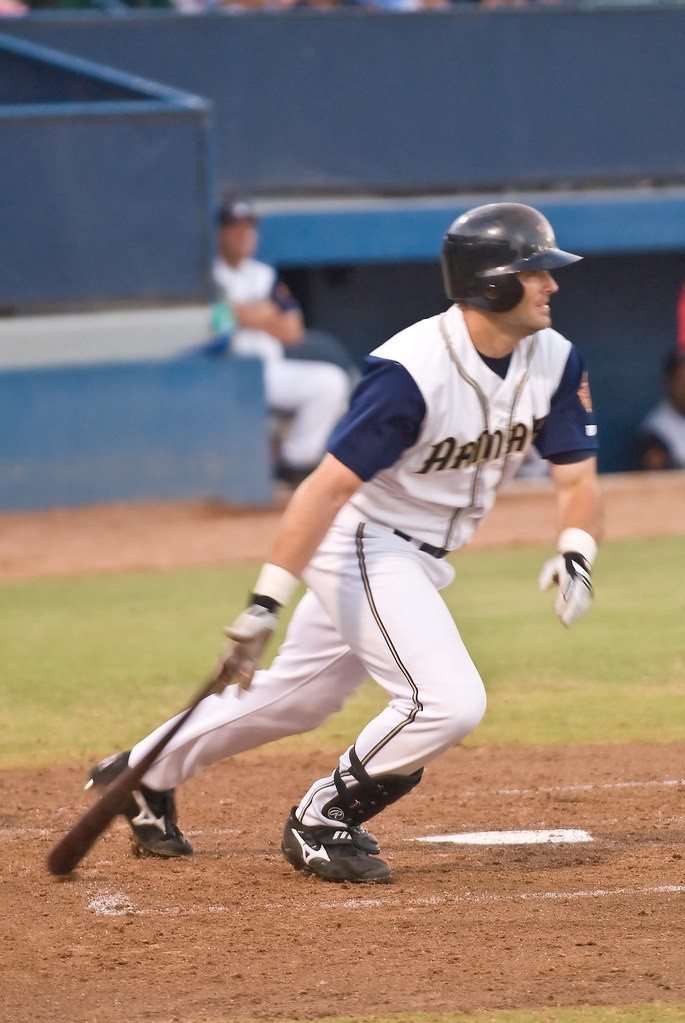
[634,348,685,472]
[89,201,606,881]
[209,200,358,482]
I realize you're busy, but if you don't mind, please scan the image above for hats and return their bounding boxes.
[218,198,262,223]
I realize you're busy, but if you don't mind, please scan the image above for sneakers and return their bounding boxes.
[278,805,390,882]
[84,751,195,856]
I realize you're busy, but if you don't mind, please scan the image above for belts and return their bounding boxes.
[393,527,450,560]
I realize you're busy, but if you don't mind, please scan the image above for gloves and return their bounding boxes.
[537,526,598,628]
[213,563,301,690]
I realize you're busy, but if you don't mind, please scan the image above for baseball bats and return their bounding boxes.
[48,654,237,876]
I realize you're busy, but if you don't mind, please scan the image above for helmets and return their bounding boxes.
[442,202,584,313]
